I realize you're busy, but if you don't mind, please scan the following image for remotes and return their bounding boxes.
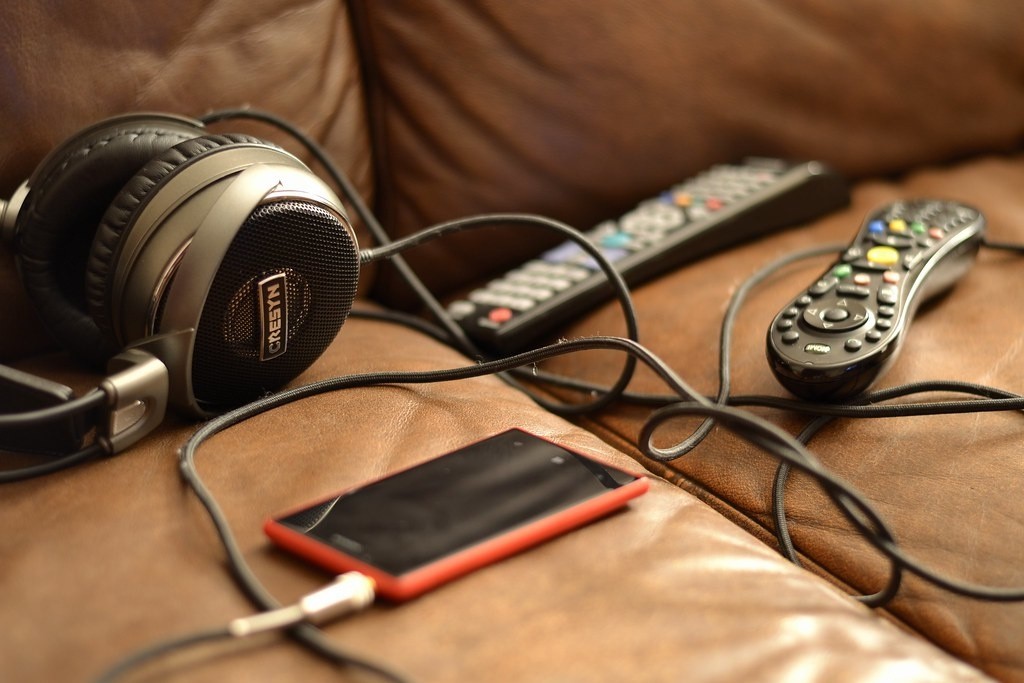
[766,199,988,401]
[446,160,852,354]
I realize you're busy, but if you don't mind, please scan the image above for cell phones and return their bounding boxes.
[261,425,651,605]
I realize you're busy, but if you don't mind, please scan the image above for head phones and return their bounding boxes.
[0,109,363,480]
